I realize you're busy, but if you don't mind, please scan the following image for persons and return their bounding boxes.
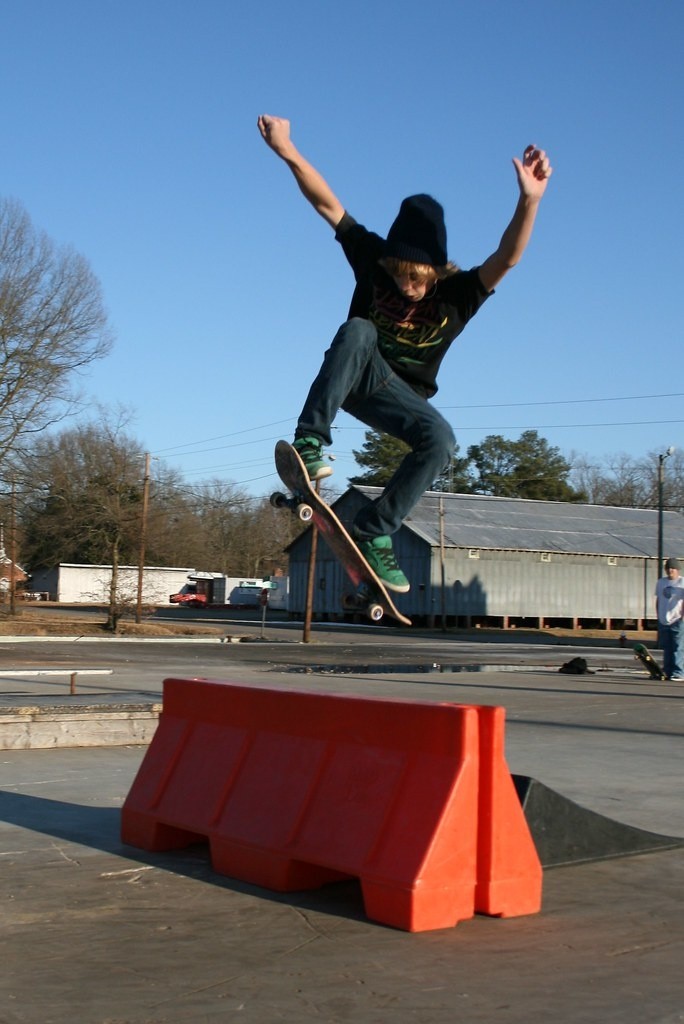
[256,114,551,592]
[655,557,684,680]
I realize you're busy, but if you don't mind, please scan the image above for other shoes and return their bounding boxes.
[657,672,668,678]
[669,676,684,682]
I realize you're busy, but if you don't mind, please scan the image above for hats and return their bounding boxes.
[386,193,447,267]
[665,557,680,569]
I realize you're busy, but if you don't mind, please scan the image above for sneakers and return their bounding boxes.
[355,536,411,593]
[292,438,333,480]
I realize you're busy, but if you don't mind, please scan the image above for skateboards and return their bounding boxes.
[633,643,669,681]
[270,439,414,626]
